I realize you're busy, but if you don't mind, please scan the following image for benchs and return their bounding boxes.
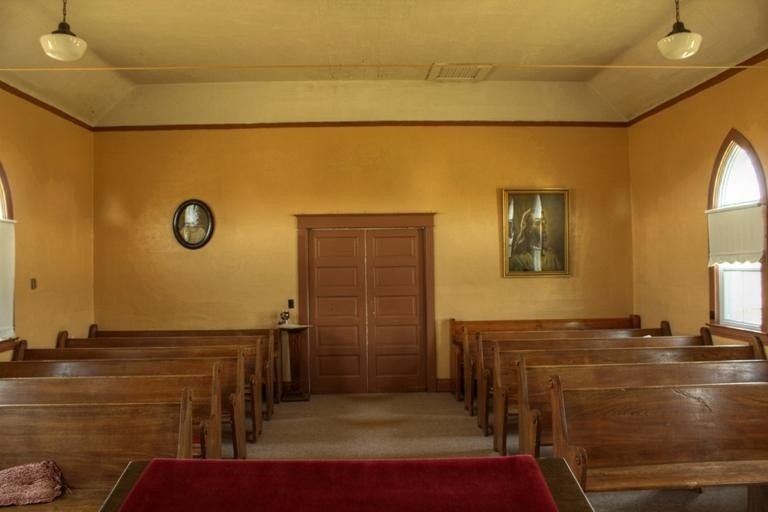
[10,340,246,458]
[54,330,266,442]
[516,349,768,458]
[0,387,194,511]
[2,358,222,461]
[448,314,714,456]
[86,324,283,421]
[549,366,768,498]
[490,336,765,451]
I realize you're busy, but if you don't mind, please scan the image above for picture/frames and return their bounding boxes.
[499,183,573,280]
[168,198,219,250]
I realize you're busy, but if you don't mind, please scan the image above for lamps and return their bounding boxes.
[36,1,91,65]
[650,1,711,61]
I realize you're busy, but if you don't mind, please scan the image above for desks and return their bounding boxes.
[96,450,594,511]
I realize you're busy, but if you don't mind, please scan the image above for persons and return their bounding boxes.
[179,205,208,244]
[509,207,564,271]
